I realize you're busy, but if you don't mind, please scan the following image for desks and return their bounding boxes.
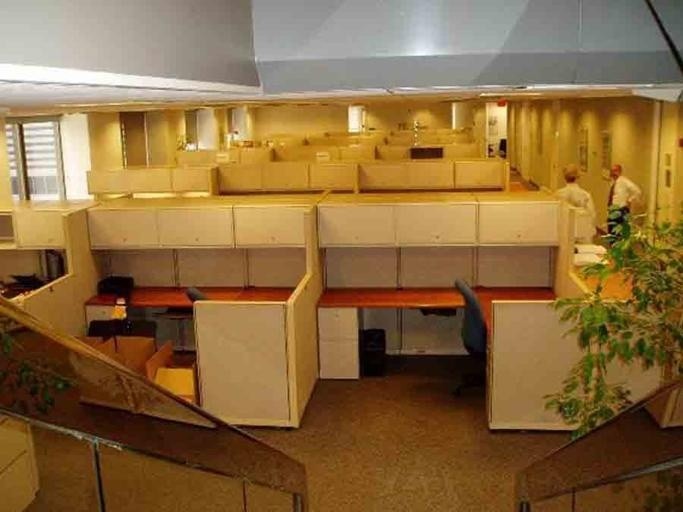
[316,286,559,379]
[82,285,300,337]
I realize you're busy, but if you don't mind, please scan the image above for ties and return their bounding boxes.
[607,181,615,205]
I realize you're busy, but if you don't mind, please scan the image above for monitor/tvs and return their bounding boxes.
[410,147,443,159]
[44,249,64,283]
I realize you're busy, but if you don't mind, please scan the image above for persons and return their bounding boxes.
[605,164,644,249]
[554,161,596,241]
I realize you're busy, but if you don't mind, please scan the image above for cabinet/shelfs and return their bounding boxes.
[215,126,509,191]
[0,198,109,287]
[175,148,208,166]
[85,193,321,289]
[315,184,561,289]
[86,165,217,192]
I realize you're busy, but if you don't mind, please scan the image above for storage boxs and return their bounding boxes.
[92,336,156,378]
[69,333,102,349]
[144,338,198,409]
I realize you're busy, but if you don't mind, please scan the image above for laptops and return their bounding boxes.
[7,274,45,290]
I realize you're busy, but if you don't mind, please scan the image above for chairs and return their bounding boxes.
[184,286,207,301]
[446,277,487,395]
